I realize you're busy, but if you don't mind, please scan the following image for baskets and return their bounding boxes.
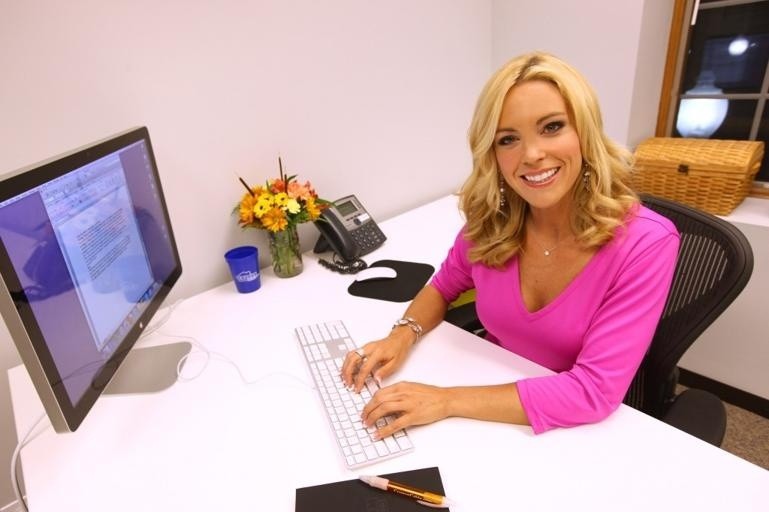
[630,136,765,217]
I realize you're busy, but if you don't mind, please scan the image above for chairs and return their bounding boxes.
[622,194,756,446]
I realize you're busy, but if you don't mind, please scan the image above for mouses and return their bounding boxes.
[355,266,398,282]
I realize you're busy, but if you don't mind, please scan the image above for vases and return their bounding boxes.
[266,226,305,278]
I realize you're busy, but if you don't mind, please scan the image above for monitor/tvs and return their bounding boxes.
[0,126,183,433]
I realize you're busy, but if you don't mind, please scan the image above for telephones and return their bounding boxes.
[316,194,387,267]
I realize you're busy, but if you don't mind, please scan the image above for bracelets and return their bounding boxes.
[392,318,423,344]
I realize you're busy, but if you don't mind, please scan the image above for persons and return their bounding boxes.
[340,48,682,441]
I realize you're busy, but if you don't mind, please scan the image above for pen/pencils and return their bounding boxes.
[357,475,452,508]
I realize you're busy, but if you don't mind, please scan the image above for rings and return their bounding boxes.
[356,348,366,358]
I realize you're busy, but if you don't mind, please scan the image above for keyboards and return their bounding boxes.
[295,320,415,470]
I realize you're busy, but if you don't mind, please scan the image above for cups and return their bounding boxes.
[224,246,262,294]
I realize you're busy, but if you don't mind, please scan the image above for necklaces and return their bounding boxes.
[527,231,575,257]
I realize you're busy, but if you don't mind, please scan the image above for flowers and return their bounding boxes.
[230,155,335,233]
